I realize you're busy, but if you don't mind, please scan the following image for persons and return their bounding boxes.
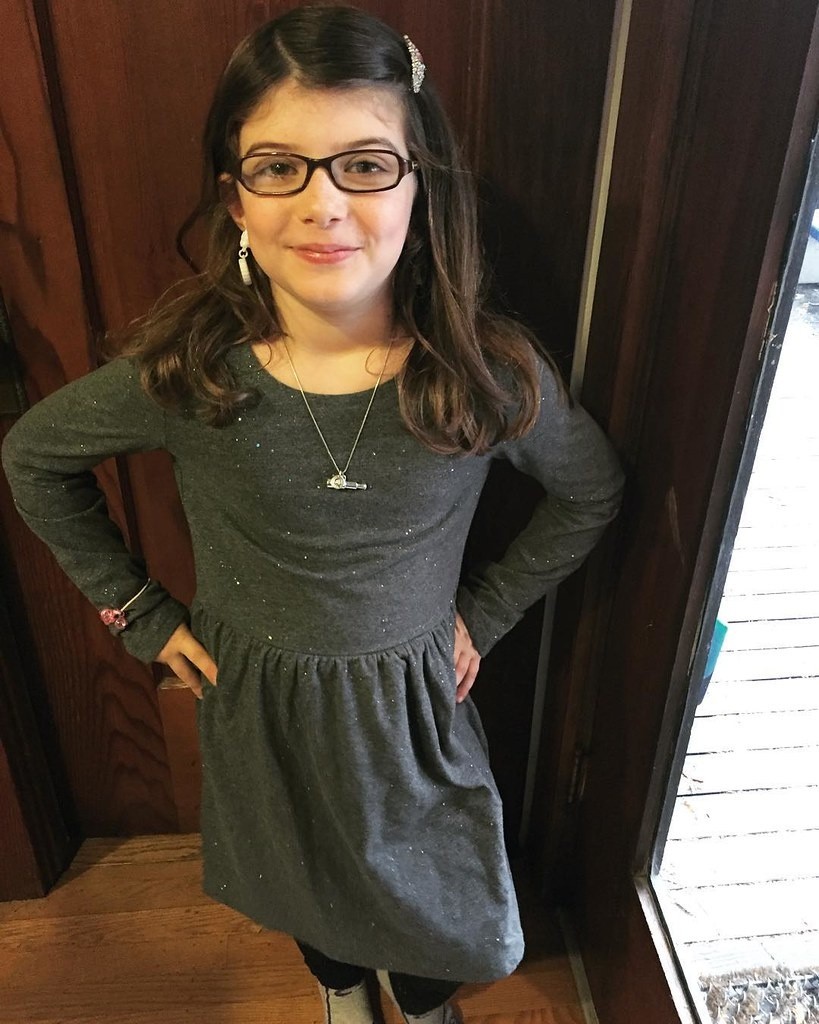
[0,10,629,1024]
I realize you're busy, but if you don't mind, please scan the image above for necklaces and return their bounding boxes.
[283,336,393,490]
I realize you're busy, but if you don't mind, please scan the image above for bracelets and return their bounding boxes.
[101,577,148,629]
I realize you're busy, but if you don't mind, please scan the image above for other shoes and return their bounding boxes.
[316,980,375,1024]
[375,968,459,1024]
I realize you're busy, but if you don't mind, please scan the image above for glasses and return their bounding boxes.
[228,146,418,195]
